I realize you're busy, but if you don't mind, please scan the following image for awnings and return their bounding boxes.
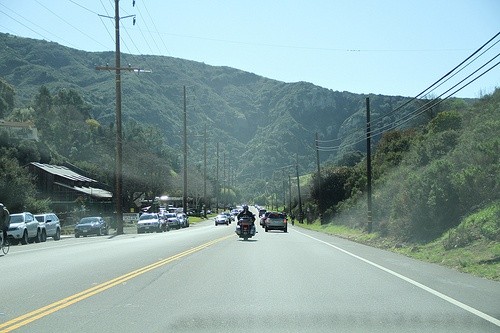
[55,182,114,198]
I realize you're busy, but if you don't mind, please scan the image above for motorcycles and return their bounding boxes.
[235,214,258,241]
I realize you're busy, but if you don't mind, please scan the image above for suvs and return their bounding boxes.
[0,212,41,245]
[33,213,61,242]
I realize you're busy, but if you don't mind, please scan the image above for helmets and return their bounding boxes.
[243,205,248,209]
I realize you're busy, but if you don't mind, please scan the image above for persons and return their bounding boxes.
[0,203,10,246]
[237,206,255,225]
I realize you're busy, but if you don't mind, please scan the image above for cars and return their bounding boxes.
[214,209,243,225]
[264,211,288,232]
[254,203,271,225]
[136,206,212,233]
[74,216,108,238]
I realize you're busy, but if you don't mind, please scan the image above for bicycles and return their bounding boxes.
[0,228,10,255]
